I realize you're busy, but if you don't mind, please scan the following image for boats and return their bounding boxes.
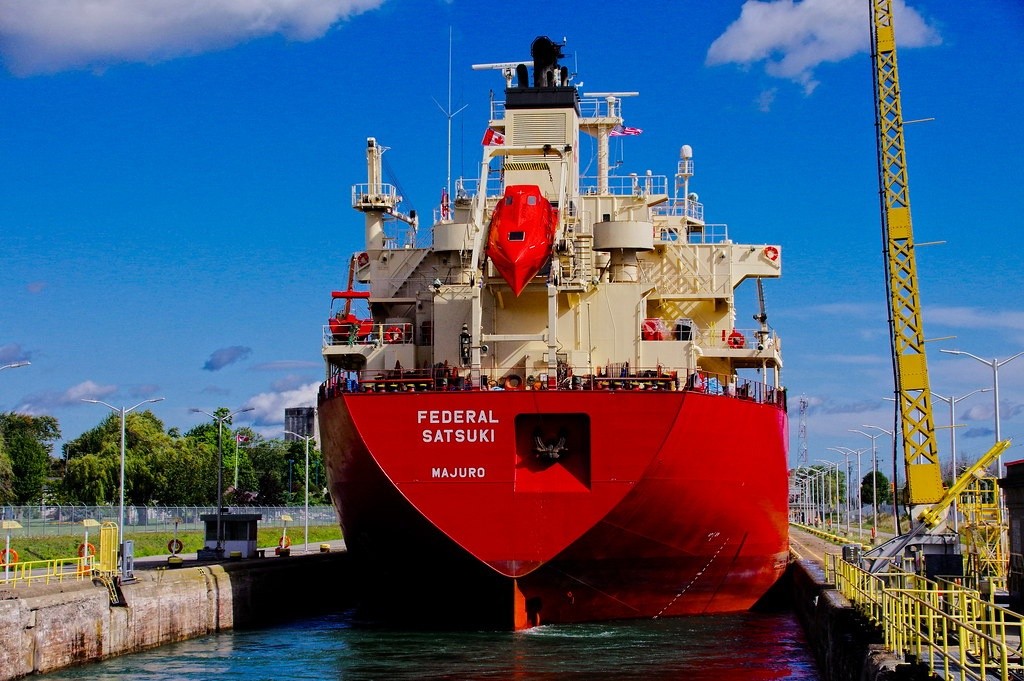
[485,185,559,298]
[314,24,789,632]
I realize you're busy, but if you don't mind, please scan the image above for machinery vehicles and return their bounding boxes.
[859,1,1013,607]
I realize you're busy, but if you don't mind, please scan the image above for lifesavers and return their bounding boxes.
[386,326,402,343]
[764,246,778,261]
[168,539,183,554]
[78,543,95,560]
[0,548,18,568]
[357,253,370,267]
[728,332,745,349]
[279,536,291,548]
[504,374,524,390]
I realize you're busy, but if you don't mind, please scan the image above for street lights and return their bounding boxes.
[826,446,876,538]
[917,387,994,555]
[848,429,888,537]
[280,429,321,553]
[940,348,1024,522]
[794,459,847,530]
[191,408,256,549]
[862,425,905,539]
[81,398,166,549]
